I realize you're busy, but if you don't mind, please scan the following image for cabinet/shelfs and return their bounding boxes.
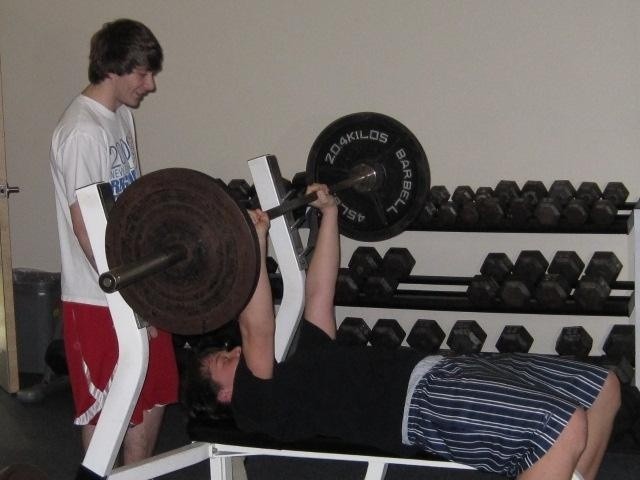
[268,195,635,401]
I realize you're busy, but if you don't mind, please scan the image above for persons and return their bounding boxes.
[180,181,623,480]
[48,19,181,466]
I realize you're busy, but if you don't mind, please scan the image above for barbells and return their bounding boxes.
[99,111,432,336]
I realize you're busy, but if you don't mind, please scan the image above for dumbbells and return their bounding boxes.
[334,246,416,307]
[336,317,635,386]
[418,180,629,226]
[466,251,623,311]
[215,171,308,219]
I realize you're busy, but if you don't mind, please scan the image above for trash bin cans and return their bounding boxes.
[11,270,64,372]
